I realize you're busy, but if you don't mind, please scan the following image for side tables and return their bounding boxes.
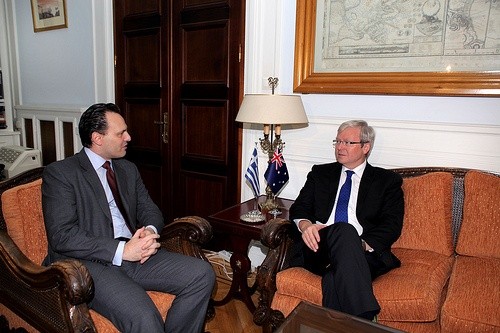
[209,196,296,312]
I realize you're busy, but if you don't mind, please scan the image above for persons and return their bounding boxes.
[41,102,215,333]
[287,120,405,324]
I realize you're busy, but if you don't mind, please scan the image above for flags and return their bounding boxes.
[264,144,289,197]
[245,146,261,200]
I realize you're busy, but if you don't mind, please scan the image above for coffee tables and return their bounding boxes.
[274,301,408,333]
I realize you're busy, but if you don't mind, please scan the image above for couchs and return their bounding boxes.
[0,166,217,333]
[254,165,500,333]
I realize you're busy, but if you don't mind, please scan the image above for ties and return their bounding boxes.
[102,161,135,234]
[334,170,355,223]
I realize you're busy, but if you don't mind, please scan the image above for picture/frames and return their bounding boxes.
[30,0,68,33]
[293,0,500,96]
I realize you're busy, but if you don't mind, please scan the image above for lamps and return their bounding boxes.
[236,76,308,210]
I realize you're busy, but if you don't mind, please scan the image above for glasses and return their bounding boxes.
[333,140,364,146]
